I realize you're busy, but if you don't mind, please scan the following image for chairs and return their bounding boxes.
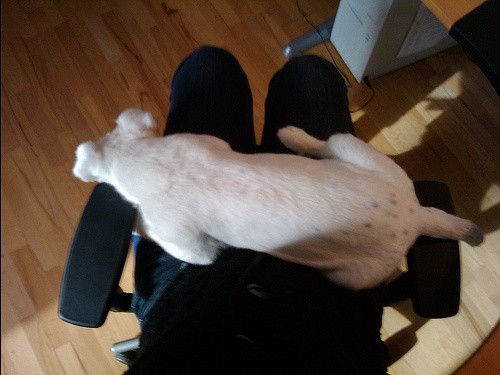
[58,172,461,328]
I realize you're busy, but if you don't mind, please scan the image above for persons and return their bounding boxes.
[119,43,392,375]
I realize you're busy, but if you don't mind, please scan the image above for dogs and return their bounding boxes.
[72,107,484,293]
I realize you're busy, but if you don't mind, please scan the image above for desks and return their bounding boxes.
[281,0,500,102]
